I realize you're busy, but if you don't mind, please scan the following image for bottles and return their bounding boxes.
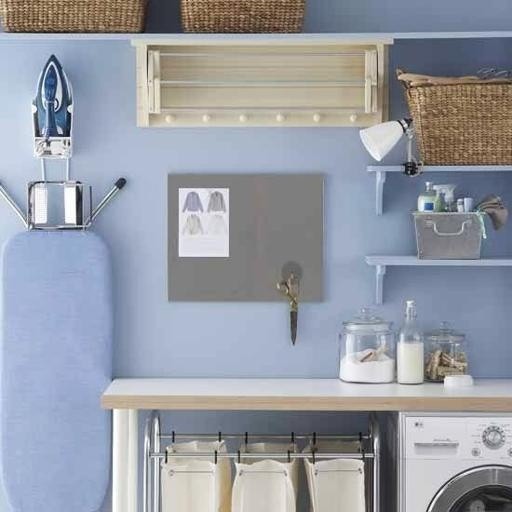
[417,181,440,212]
[341,300,469,384]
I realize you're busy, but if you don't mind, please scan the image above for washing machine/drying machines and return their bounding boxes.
[405,416,512,512]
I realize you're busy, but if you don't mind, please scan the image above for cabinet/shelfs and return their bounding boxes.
[367,164,511,309]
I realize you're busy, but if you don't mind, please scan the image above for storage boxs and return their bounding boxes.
[410,210,486,260]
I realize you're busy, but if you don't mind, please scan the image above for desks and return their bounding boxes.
[102,377,508,511]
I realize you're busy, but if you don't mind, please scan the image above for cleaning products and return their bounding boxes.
[432,184,459,212]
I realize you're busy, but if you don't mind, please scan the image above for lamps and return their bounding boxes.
[357,117,422,178]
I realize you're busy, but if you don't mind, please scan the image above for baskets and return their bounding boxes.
[405,83,512,165]
[181,0,304,33]
[0,0,148,33]
